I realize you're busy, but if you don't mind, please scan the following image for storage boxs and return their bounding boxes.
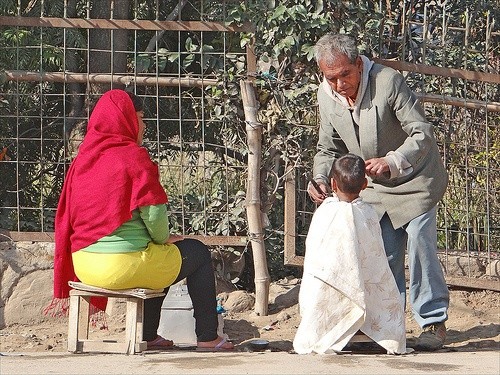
[157,285,224,347]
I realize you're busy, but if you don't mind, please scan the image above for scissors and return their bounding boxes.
[302,174,327,201]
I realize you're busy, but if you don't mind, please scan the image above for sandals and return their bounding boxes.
[195,337,236,352]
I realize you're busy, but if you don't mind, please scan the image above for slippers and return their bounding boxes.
[142,334,175,349]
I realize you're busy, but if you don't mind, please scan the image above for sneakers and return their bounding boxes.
[414,321,447,350]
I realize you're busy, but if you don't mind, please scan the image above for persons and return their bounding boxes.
[292,153,406,355]
[53,88,238,352]
[306,32,450,351]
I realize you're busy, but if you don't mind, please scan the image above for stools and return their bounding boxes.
[67,290,147,355]
